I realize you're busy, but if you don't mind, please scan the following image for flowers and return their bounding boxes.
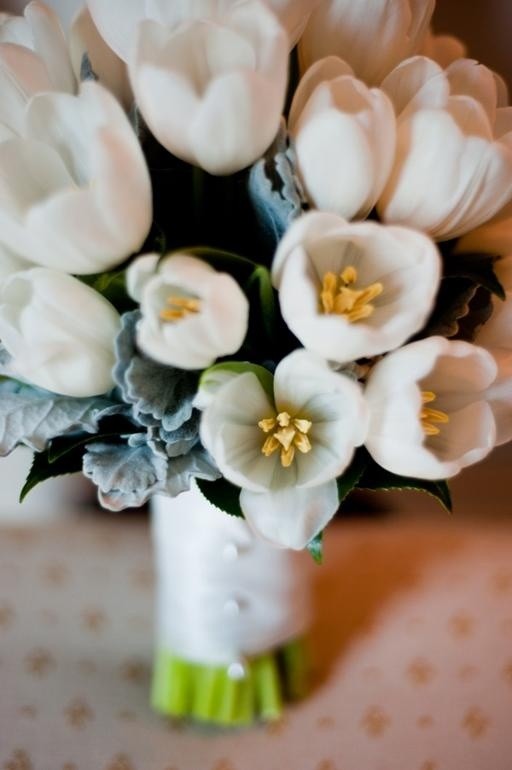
[0,1,512,737]
